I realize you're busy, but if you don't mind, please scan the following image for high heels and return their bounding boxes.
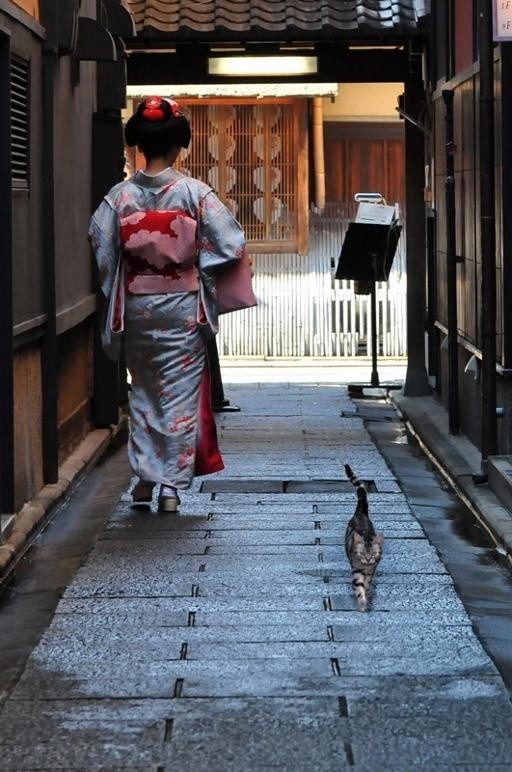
[128,488,179,512]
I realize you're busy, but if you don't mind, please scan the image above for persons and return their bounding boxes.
[86,93,258,517]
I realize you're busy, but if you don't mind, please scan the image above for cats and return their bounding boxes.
[344,463,384,613]
